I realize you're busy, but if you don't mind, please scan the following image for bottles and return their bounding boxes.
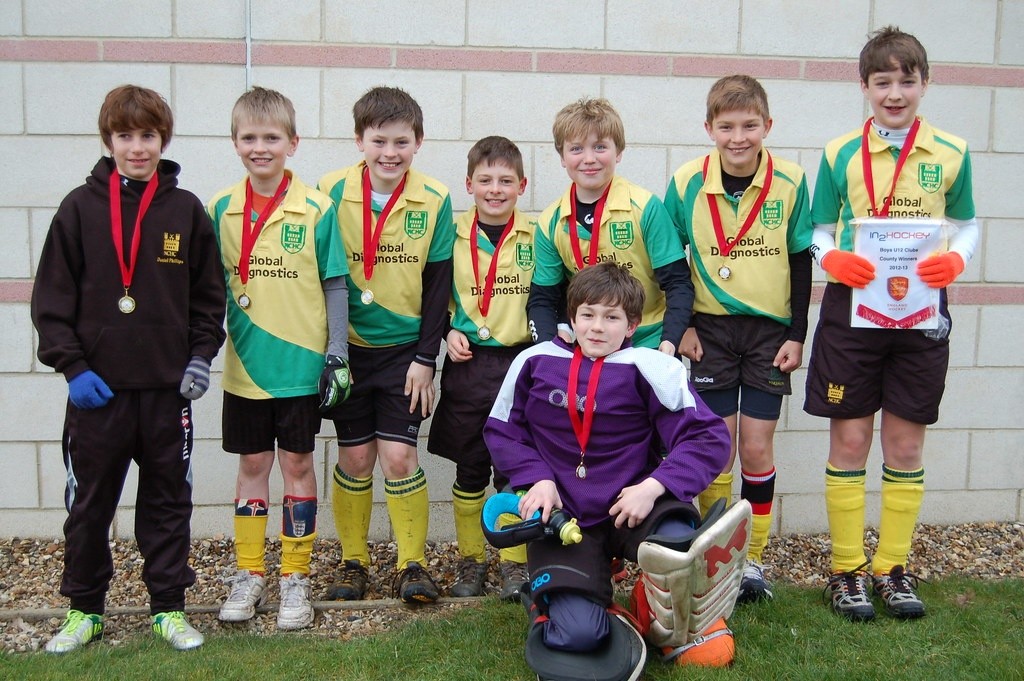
[516,490,582,544]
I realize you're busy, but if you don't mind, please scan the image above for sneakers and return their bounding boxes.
[499,559,529,601]
[219,569,269,621]
[277,571,314,629]
[392,560,439,604]
[46,610,105,653]
[327,559,369,601]
[871,565,931,619]
[822,560,876,622]
[149,611,204,649]
[736,558,773,604]
[451,556,487,597]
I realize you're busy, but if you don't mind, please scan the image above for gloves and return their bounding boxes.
[318,354,351,407]
[916,252,964,288]
[821,250,875,289]
[180,356,210,399]
[69,370,114,408]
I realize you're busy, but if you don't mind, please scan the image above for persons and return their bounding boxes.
[483,262,752,681]
[665,75,813,605]
[30,86,227,650]
[427,136,537,596]
[316,87,455,601]
[811,27,979,621]
[535,100,692,358]
[205,87,351,630]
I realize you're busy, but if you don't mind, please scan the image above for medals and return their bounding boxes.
[477,325,491,341]
[238,293,250,309]
[361,289,374,304]
[119,297,135,313]
[576,464,587,479]
[718,267,731,280]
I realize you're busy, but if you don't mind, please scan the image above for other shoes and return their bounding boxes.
[611,557,627,581]
